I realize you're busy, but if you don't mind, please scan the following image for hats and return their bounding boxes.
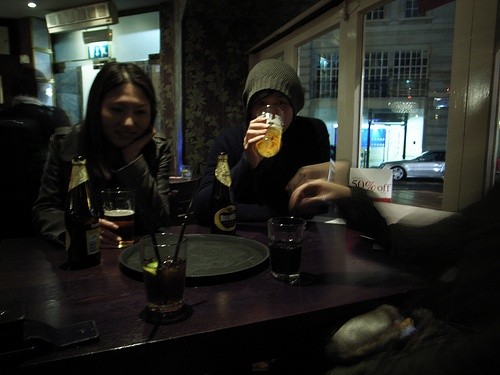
[242,58,304,115]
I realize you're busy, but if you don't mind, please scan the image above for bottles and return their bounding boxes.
[211,152,237,236]
[65,155,102,269]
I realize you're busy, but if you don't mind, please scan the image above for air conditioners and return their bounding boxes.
[82,26,112,44]
[45,1,118,34]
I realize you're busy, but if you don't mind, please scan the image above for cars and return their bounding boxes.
[379,150,447,179]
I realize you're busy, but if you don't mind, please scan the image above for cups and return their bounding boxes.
[256,105,285,157]
[269,216,305,284]
[102,189,135,247]
[141,234,191,311]
[182,166,192,180]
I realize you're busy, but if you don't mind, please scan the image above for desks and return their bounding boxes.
[0,225,452,375]
[167,174,200,224]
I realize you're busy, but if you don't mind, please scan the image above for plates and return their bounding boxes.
[119,236,270,280]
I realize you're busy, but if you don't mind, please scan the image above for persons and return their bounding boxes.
[289,178,500,375]
[36,62,173,248]
[192,58,331,227]
